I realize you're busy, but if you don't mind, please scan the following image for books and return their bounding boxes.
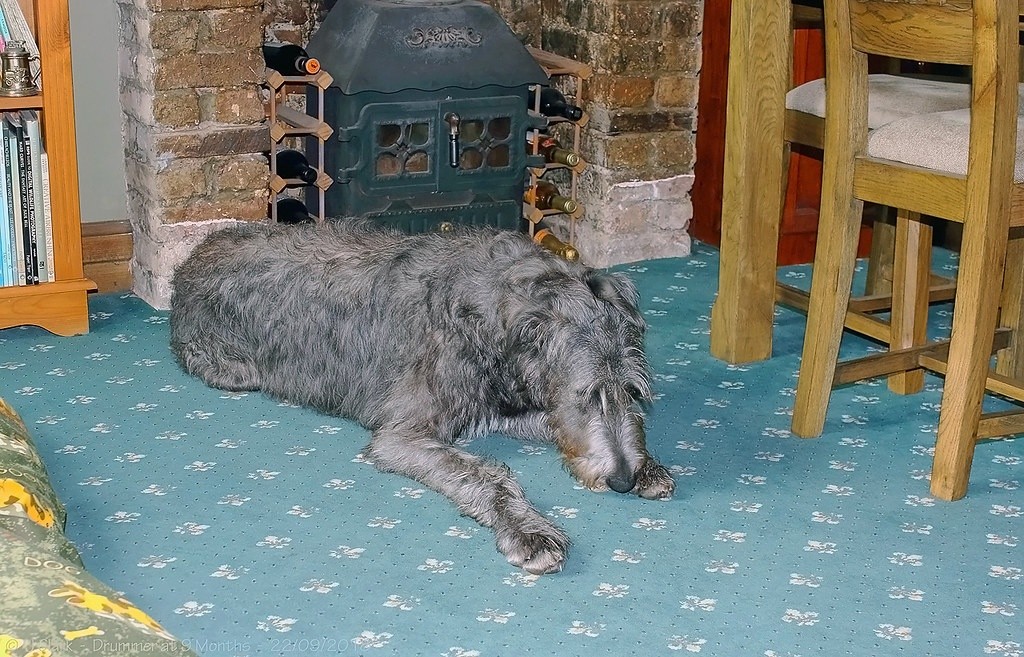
[0,110,56,290]
[0,0,42,93]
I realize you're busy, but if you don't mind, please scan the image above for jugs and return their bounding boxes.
[0,40,41,98]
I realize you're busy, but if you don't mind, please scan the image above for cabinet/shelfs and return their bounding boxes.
[0,0,98,335]
[688,0,878,267]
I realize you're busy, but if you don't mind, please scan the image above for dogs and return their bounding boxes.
[169,215,676,577]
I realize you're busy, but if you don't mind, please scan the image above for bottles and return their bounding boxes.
[522,179,578,214]
[262,41,321,76]
[528,83,584,121]
[525,131,580,167]
[521,219,580,263]
[267,193,315,225]
[263,143,318,183]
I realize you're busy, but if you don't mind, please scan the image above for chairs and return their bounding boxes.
[774,0,973,394]
[790,0,1024,502]
